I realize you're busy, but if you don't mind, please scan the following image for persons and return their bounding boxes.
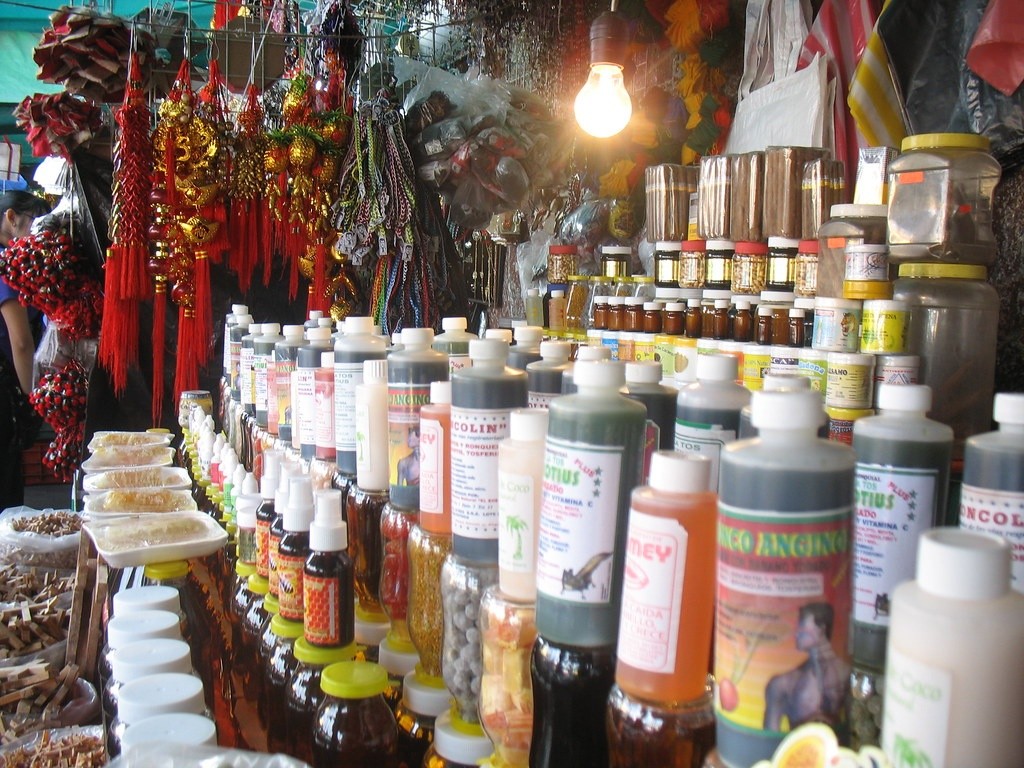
[0,188,52,509]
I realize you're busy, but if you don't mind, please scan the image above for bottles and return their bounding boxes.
[100,237,1023,768]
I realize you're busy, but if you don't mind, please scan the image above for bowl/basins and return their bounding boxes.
[82,431,229,569]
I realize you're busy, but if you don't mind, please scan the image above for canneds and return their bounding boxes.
[541,235,919,445]
